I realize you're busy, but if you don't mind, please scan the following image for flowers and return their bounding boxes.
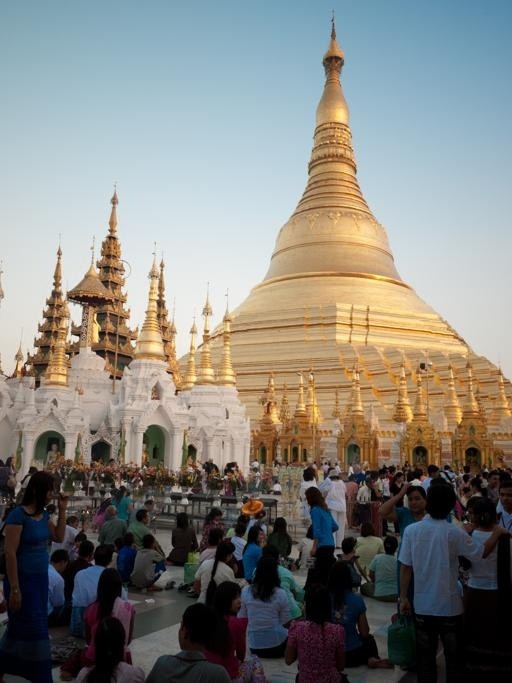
[51,452,238,505]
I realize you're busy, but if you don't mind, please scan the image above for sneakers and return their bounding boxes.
[368,658,394,669]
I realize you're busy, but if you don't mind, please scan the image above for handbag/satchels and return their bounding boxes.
[387,597,417,668]
[305,522,314,540]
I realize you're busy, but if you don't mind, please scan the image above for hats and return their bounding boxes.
[242,498,264,515]
[328,470,339,477]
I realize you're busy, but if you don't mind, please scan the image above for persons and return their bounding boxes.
[0,454,511,683]
